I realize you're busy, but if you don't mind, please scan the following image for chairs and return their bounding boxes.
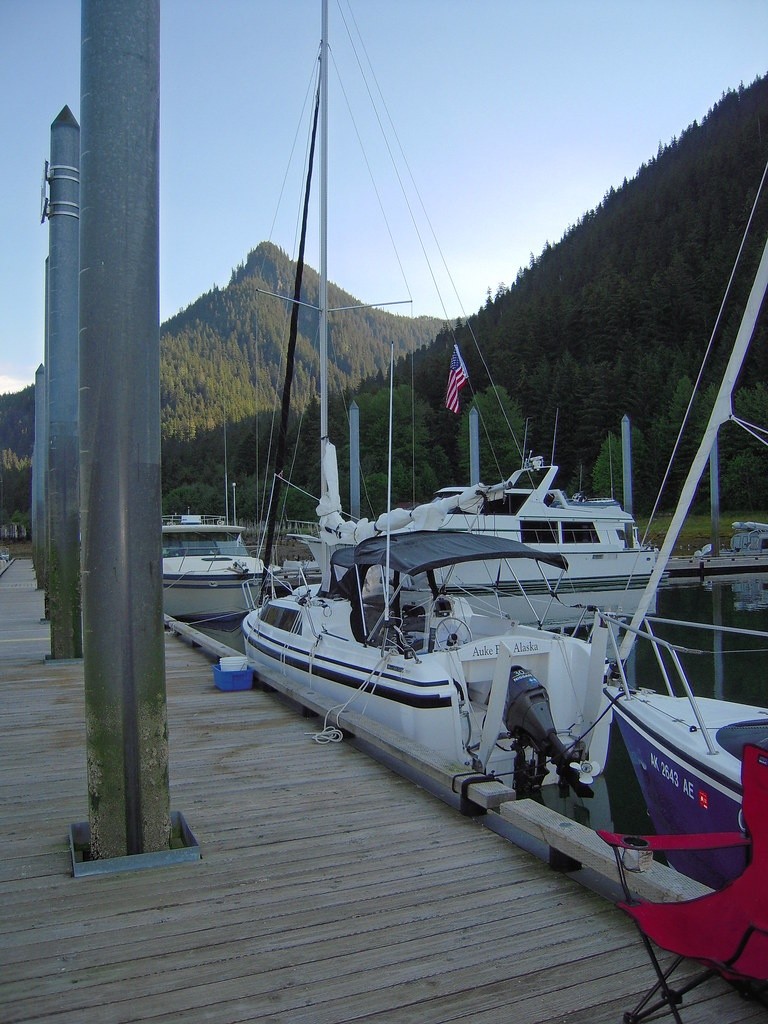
[595,744,768,1024]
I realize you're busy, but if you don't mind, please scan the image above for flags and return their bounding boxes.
[444,343,469,416]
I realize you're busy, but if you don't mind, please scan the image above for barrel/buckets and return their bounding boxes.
[219,657,248,671]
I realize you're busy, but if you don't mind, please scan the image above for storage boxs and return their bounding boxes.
[212,665,259,690]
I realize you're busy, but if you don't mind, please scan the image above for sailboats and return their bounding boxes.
[606,158,768,889]
[239,0,615,801]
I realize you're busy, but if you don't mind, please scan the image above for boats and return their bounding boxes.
[159,514,277,625]
[287,403,768,589]
